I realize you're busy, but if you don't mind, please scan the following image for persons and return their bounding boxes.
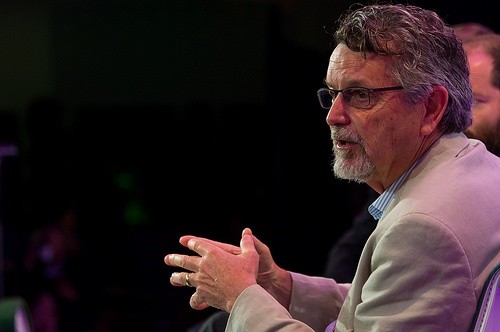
[164,4,500,332]
[326,25,500,281]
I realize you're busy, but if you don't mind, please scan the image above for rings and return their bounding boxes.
[185,273,192,288]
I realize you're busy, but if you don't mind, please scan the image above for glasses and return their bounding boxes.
[317,85,406,109]
